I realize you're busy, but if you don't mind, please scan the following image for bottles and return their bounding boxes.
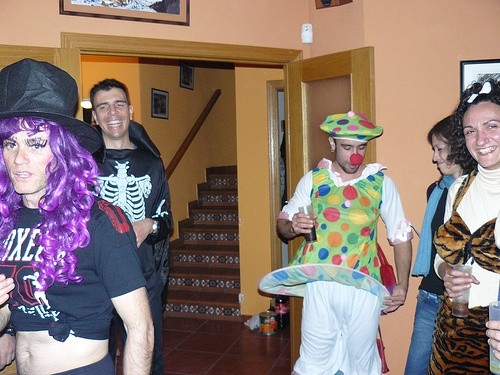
[269,294,290,332]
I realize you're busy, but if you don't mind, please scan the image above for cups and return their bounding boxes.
[298,204,317,245]
[452,264,473,318]
[489,302,500,375]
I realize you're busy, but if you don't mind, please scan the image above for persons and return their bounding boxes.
[427,75,500,375]
[257,108,415,375]
[89,77,174,375]
[0,57,155,375]
[0,323,18,373]
[404,114,475,375]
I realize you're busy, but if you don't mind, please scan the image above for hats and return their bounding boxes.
[0,58,103,154]
[320,110,383,143]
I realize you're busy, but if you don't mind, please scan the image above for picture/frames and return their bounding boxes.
[460,58,500,95]
[151,88,169,119]
[59,0,190,27]
[179,61,194,90]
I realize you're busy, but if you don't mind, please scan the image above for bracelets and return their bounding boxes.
[3,324,17,336]
[398,281,409,291]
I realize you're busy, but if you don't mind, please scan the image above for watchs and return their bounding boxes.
[151,219,160,235]
